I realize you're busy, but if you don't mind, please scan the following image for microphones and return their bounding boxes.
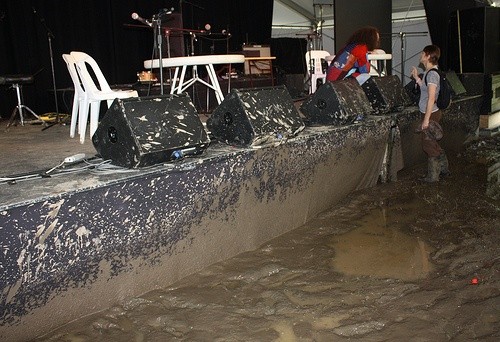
[205,24,211,34]
[222,29,232,36]
[132,13,150,25]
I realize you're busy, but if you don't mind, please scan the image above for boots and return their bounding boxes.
[415,149,448,185]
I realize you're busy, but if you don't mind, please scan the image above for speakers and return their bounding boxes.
[299,76,374,126]
[445,71,467,100]
[206,83,306,147]
[92,92,211,168]
[361,75,413,116]
[404,72,424,107]
[242,44,271,75]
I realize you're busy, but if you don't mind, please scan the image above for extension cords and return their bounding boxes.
[64,152,85,162]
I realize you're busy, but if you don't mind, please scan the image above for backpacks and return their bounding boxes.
[424,68,451,109]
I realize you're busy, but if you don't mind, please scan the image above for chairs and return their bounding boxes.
[71,51,139,145]
[306,49,332,94]
[61,53,115,140]
[368,49,388,76]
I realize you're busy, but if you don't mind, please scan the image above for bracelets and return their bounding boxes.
[416,78,420,83]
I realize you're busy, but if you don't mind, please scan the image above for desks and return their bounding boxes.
[143,55,245,106]
[244,57,277,88]
[325,54,392,77]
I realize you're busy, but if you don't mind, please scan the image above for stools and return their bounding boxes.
[0,73,48,130]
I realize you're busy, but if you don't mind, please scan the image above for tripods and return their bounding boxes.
[31,4,88,132]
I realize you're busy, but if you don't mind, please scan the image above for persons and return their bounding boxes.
[326,27,380,82]
[410,45,449,183]
